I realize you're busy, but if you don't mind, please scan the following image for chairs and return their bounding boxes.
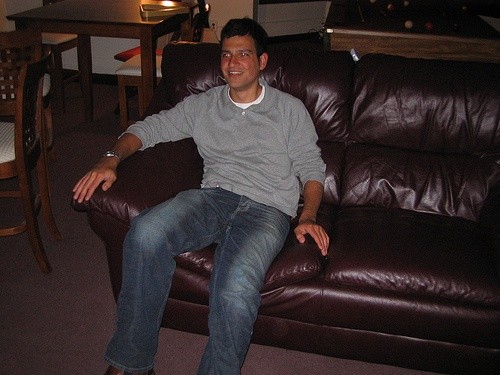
[0,26,62,273]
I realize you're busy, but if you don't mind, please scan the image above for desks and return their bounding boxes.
[324,0,500,65]
[6,0,200,128]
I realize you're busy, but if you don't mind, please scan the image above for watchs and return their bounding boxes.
[102,150,122,161]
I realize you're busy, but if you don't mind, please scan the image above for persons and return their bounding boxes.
[73,17,329,375]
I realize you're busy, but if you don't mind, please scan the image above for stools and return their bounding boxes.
[115,54,164,134]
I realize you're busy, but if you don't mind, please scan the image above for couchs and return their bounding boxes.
[71,33,500,375]
